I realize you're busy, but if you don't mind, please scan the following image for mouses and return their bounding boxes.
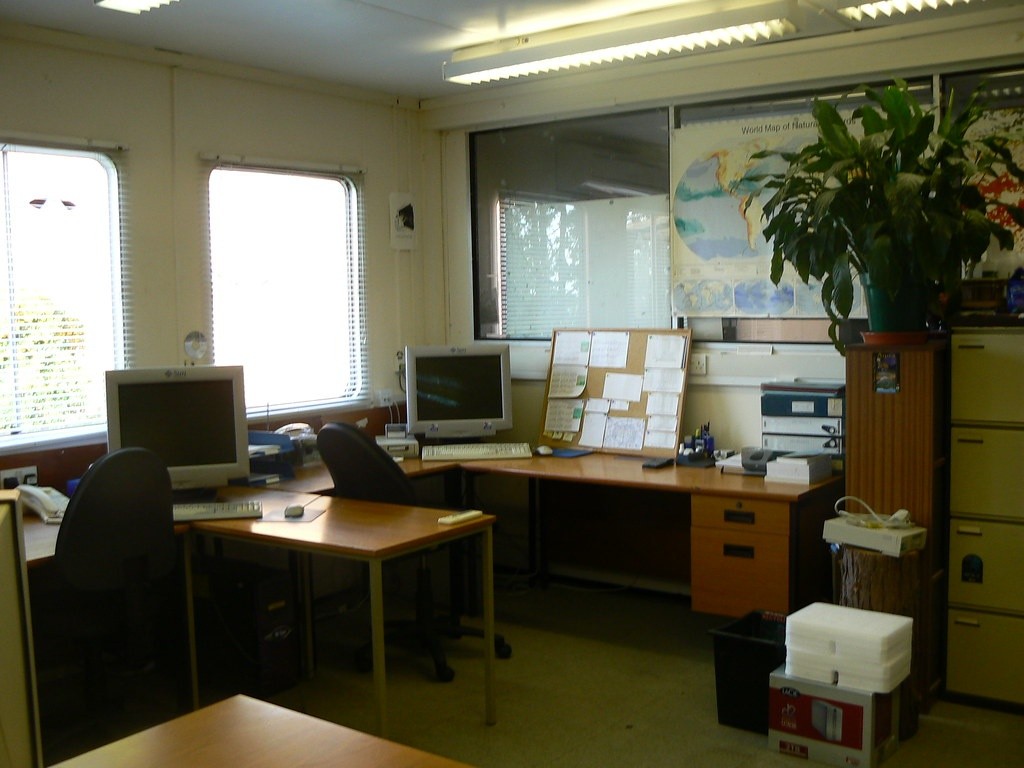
[536,446,553,455]
[284,504,304,517]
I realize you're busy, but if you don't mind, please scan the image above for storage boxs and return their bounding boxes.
[711,621,788,738]
[766,664,902,768]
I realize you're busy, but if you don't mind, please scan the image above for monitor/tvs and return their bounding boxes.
[106,366,251,504]
[404,345,513,446]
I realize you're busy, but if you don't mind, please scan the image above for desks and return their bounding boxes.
[42,692,474,768]
[0,429,844,637]
[838,339,936,563]
[192,493,497,741]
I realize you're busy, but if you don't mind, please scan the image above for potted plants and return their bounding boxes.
[728,69,1024,335]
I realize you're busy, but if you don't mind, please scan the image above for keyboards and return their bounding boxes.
[421,443,533,462]
[172,501,264,522]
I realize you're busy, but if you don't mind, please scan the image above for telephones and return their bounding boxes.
[275,422,313,438]
[17,483,69,522]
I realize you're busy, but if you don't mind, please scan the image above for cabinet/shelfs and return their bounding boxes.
[938,321,1024,710]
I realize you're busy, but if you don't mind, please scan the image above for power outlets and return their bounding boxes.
[689,353,707,379]
[0,465,39,492]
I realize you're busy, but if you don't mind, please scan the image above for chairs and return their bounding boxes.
[314,423,511,685]
[28,446,187,736]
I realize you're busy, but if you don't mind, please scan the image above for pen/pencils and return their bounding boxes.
[701,421,710,437]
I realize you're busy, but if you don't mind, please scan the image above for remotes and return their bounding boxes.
[437,510,484,526]
